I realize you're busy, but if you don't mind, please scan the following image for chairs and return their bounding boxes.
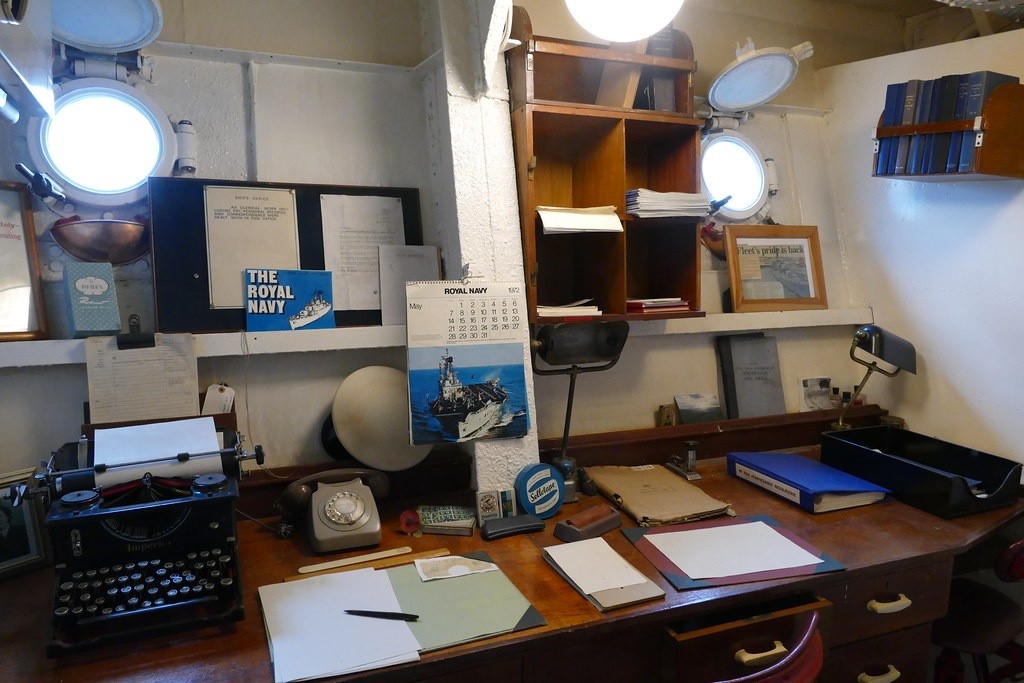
[716,610,825,683]
[930,537,1024,683]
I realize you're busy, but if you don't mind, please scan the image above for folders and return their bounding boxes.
[725,451,896,516]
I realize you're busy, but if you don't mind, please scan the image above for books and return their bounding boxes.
[628,297,690,314]
[876,70,1019,174]
[537,299,603,316]
[635,18,676,112]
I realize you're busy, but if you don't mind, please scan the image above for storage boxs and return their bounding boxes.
[659,404,674,426]
[63,262,122,339]
[822,424,1023,519]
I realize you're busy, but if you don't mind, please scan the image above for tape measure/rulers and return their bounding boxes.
[282,547,450,582]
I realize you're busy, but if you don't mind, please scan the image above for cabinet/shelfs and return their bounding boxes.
[507,5,708,320]
[0,445,1024,683]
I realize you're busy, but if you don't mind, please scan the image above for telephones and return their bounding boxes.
[275,468,396,554]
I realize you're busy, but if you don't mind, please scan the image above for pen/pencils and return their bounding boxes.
[344,609,419,620]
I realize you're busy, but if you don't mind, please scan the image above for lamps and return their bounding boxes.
[530,321,630,483]
[832,324,916,429]
[24,0,197,221]
[694,38,813,223]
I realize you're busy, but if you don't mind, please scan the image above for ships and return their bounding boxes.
[419,347,509,441]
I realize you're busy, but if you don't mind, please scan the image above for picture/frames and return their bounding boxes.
[0,467,54,578]
[724,223,829,313]
